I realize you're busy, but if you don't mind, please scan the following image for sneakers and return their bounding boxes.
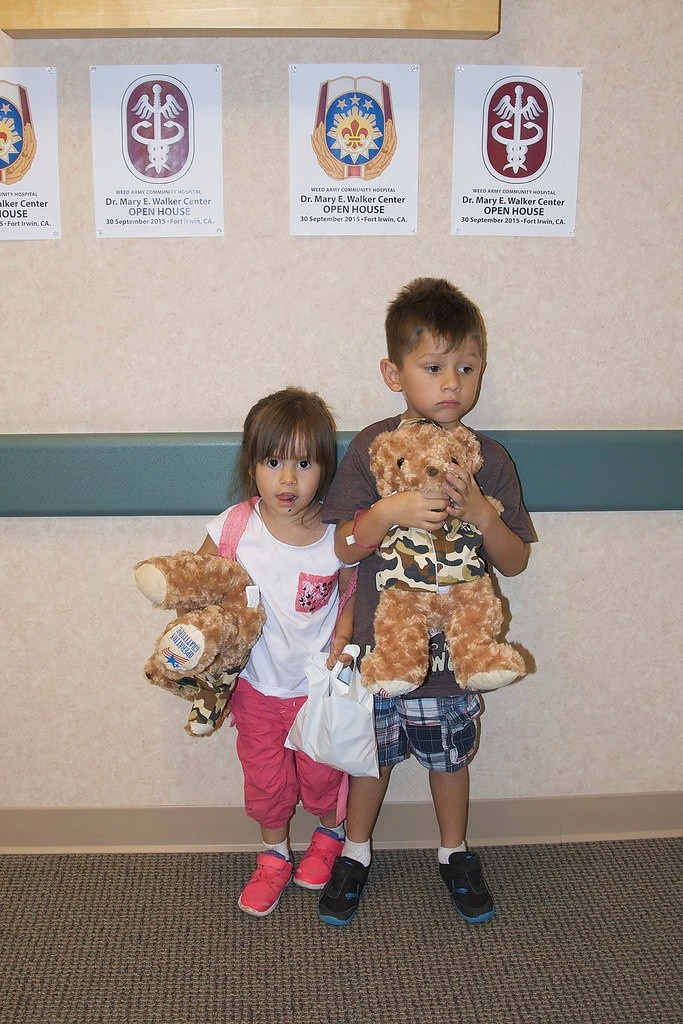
[237,851,293,918]
[294,828,347,890]
[317,855,372,925]
[438,851,494,922]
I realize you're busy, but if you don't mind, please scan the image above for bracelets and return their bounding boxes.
[352,510,381,550]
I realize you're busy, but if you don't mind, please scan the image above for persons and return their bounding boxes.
[195,388,356,917]
[317,277,537,926]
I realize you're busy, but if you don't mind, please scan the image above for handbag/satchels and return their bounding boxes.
[285,643,381,783]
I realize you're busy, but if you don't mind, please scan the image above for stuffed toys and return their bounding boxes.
[360,424,527,696]
[134,550,267,739]
